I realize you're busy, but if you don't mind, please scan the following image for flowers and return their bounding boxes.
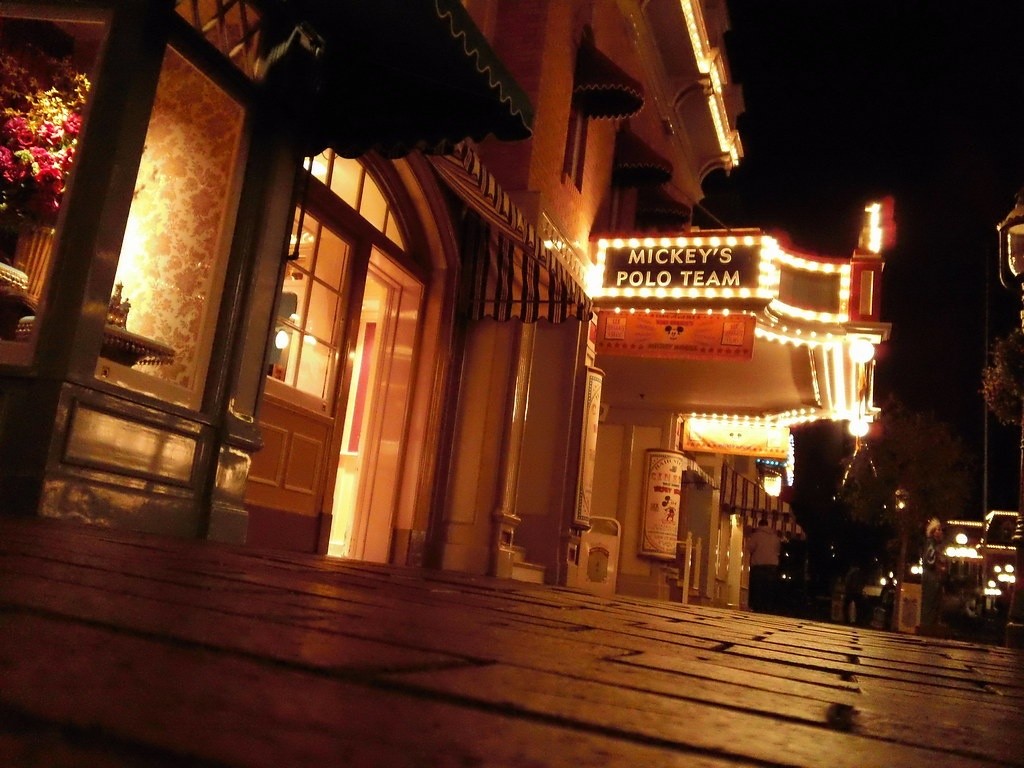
[0,68,91,221]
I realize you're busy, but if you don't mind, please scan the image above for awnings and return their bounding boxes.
[570,44,645,120]
[615,129,673,189]
[252,0,534,160]
[634,185,690,225]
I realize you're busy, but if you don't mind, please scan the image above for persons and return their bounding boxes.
[749,520,780,613]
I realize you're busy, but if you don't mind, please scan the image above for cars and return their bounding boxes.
[862,576,887,597]
